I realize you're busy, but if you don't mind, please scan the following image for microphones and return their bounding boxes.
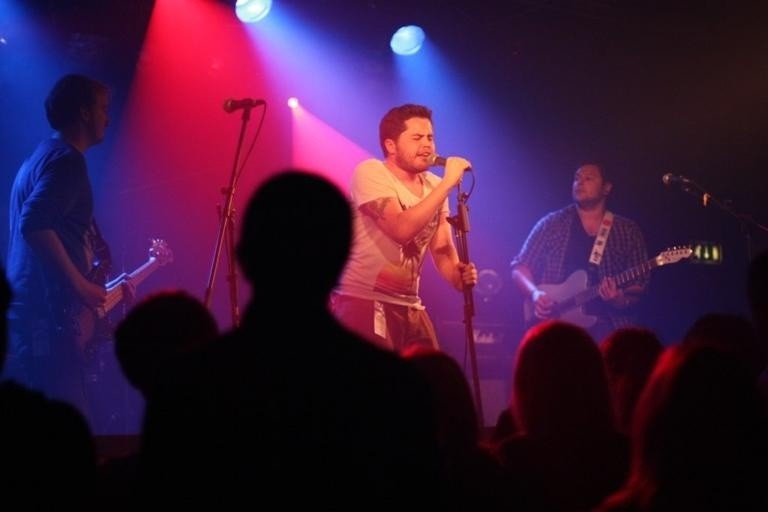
[662,172,694,193]
[223,98,264,112]
[427,153,472,171]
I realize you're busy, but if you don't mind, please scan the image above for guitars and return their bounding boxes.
[523,246,692,333]
[59,239,173,371]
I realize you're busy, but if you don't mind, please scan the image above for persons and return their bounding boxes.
[1,275,107,511]
[326,104,477,356]
[114,289,222,449]
[510,162,651,341]
[403,312,768,511]
[163,169,433,510]
[1,72,139,434]
